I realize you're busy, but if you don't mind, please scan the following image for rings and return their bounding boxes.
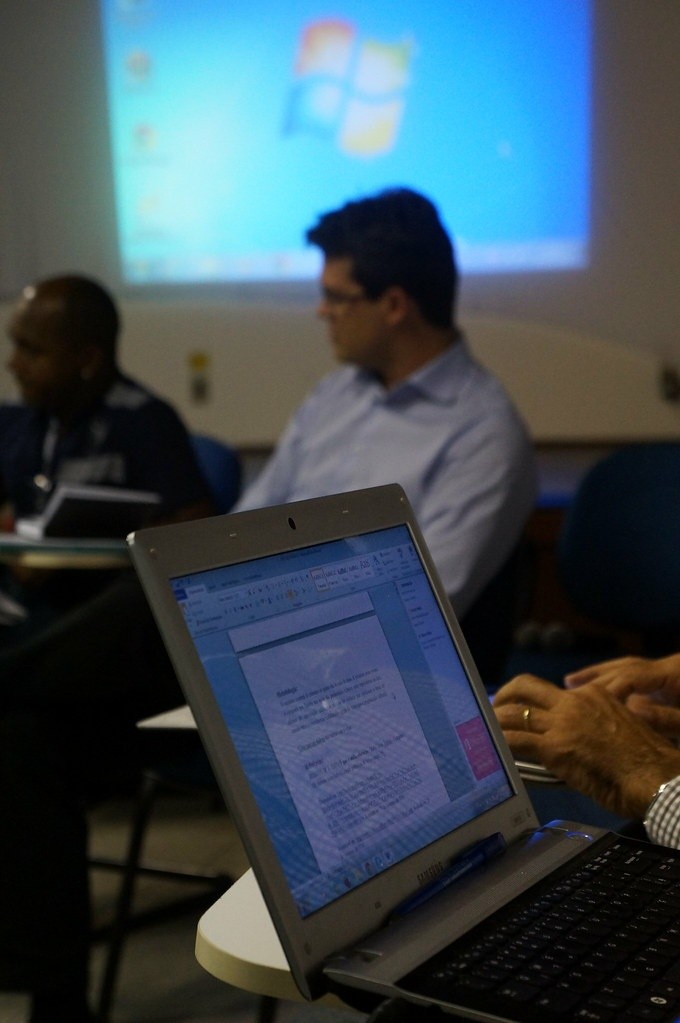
[521,699,534,734]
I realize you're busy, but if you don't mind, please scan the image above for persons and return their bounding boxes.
[484,341,680,853]
[212,181,534,634]
[0,269,202,794]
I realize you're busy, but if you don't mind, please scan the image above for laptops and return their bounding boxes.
[128,483,680,1023]
